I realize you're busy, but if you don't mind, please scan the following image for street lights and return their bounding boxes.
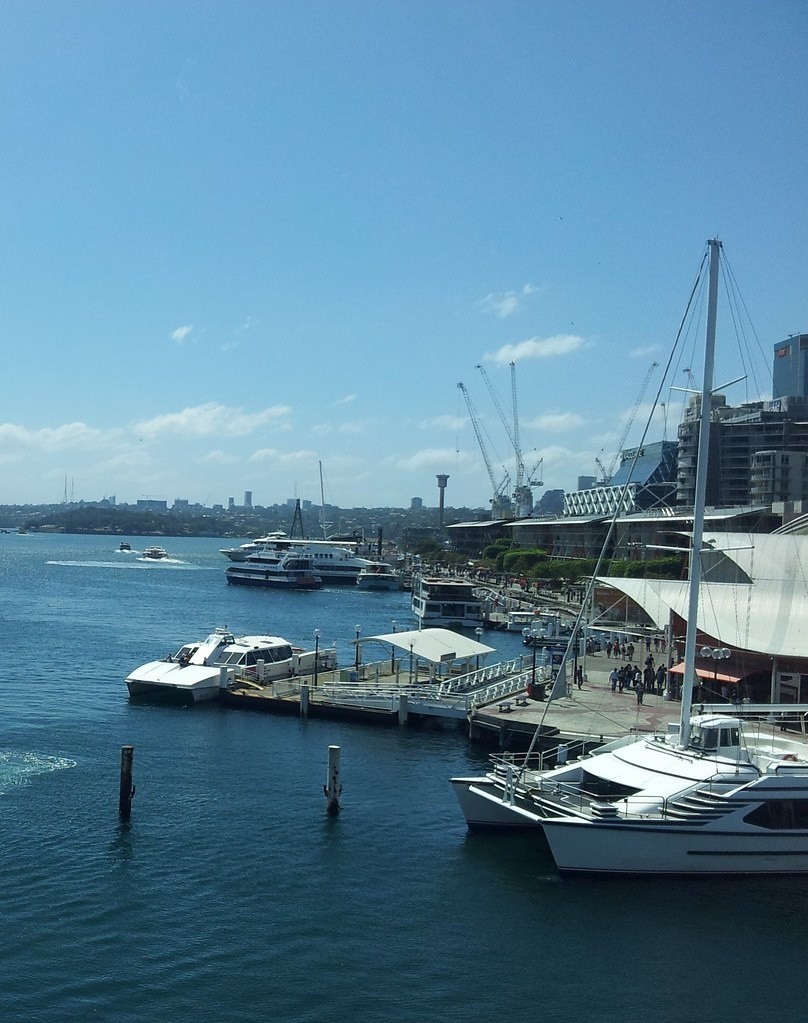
[519,627,547,686]
[353,624,362,670]
[700,644,731,704]
[391,619,397,674]
[473,627,482,672]
[313,628,322,689]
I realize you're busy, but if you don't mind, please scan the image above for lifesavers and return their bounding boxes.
[782,754,797,762]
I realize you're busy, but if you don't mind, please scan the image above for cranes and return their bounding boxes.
[526,457,544,487]
[595,457,606,482]
[456,380,511,498]
[509,361,525,489]
[683,366,699,394]
[607,360,660,484]
[477,363,530,482]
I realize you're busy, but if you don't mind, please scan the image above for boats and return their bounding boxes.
[222,539,322,589]
[356,562,399,590]
[143,545,168,559]
[506,611,549,632]
[124,623,337,704]
[519,614,589,650]
[409,578,483,629]
[447,734,654,831]
[470,237,808,883]
[273,543,369,579]
[120,541,130,551]
[221,536,267,562]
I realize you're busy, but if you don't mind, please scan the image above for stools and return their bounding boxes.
[496,701,514,713]
[513,694,529,705]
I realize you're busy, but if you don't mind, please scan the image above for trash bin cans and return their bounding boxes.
[532,684,545,701]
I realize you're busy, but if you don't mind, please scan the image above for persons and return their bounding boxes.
[586,639,591,656]
[590,637,595,656]
[595,639,607,651]
[660,635,667,654]
[609,668,618,693]
[656,666,664,695]
[576,665,584,690]
[502,590,506,596]
[623,664,632,687]
[621,643,626,661]
[648,668,656,690]
[645,634,652,652]
[166,650,214,671]
[677,656,682,665]
[667,634,673,650]
[606,641,613,658]
[635,679,644,705]
[635,669,642,684]
[627,643,635,661]
[649,653,655,668]
[645,656,652,667]
[562,618,584,636]
[344,523,607,608]
[670,676,755,711]
[699,705,705,715]
[654,635,660,652]
[631,665,642,686]
[671,657,675,668]
[625,668,633,690]
[499,590,502,595]
[613,641,619,658]
[643,666,651,687]
[660,664,667,680]
[618,666,625,693]
